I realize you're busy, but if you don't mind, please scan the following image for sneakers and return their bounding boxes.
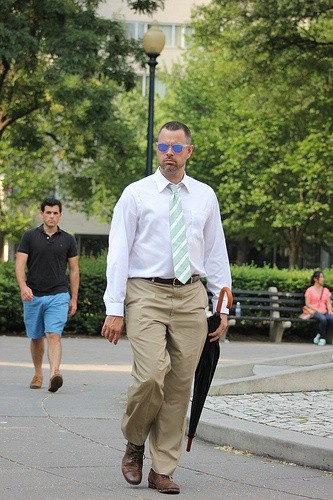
[121,442,145,485]
[148,469,180,492]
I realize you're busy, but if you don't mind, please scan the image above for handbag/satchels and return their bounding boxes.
[298,307,315,321]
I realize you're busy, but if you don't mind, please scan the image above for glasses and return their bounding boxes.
[158,144,189,153]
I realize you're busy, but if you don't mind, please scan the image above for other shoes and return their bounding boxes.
[313,333,320,344]
[318,339,326,346]
[30,376,42,389]
[48,375,63,393]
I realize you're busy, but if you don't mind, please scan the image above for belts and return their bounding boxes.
[142,274,200,287]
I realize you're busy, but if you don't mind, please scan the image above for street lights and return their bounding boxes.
[142,18,166,179]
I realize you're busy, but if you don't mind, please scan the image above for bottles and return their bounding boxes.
[235,302,241,316]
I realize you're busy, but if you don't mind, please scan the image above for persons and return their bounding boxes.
[304,271,333,346]
[101,121,232,494]
[15,198,79,393]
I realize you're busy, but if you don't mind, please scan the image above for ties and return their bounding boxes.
[168,185,191,284]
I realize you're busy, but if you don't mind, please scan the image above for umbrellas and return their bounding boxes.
[186,288,231,452]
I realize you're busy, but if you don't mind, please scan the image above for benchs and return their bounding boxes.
[206,287,333,342]
